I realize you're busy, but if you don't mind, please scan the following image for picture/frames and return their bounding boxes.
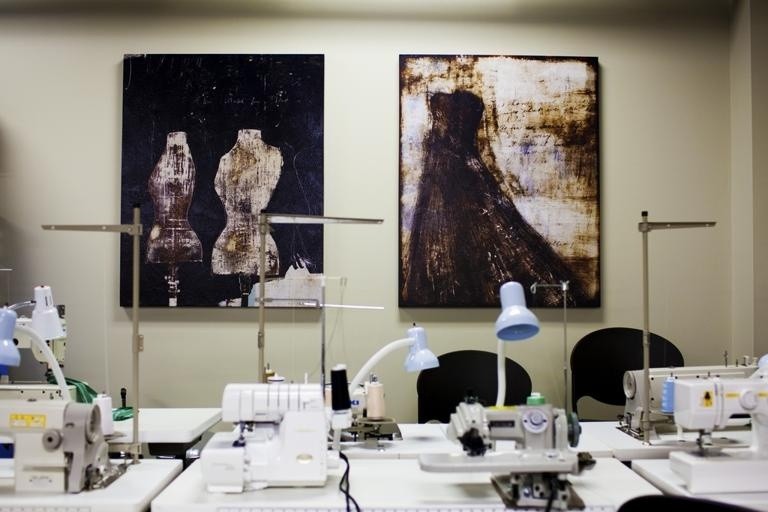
[396,53,605,309]
[120,53,326,308]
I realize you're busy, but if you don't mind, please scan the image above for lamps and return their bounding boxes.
[494,283,541,407]
[10,284,65,341]
[0,306,75,402]
[348,323,441,393]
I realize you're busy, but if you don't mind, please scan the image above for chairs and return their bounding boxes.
[418,349,532,422]
[569,327,684,423]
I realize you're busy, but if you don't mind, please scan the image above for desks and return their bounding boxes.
[0,457,181,512]
[149,456,663,512]
[106,408,222,468]
[201,422,617,458]
[631,455,768,511]
[594,420,756,462]
